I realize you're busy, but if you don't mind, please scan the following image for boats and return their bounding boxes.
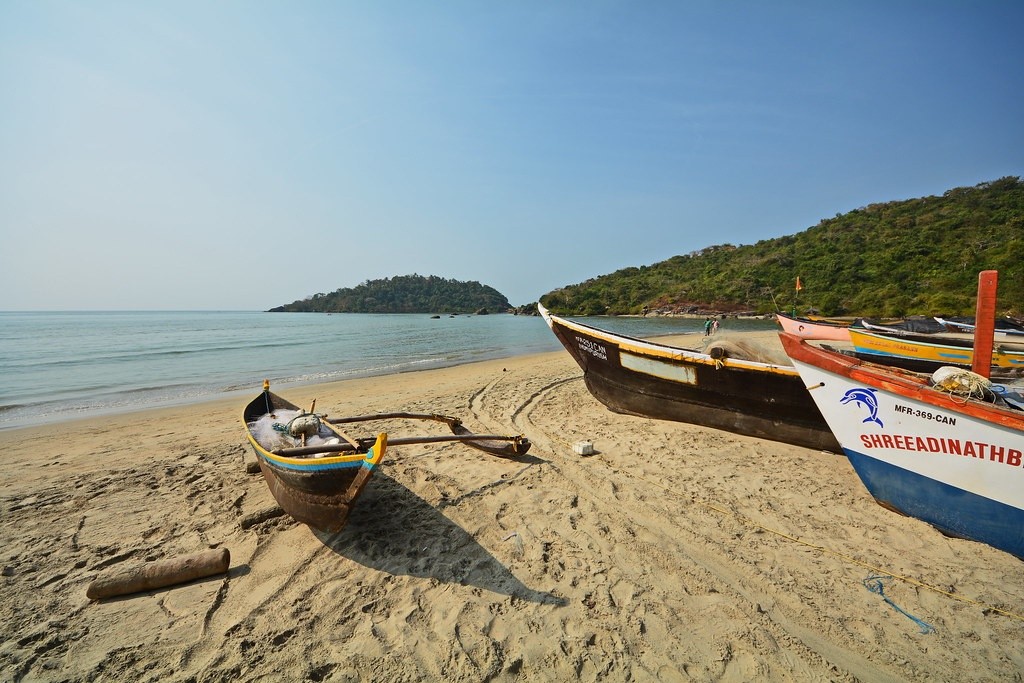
[240,377,389,535]
[775,310,1024,562]
[535,301,846,456]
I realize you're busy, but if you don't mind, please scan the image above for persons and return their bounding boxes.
[711,318,719,335]
[703,317,712,336]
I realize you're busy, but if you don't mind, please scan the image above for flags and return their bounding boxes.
[796,276,801,290]
